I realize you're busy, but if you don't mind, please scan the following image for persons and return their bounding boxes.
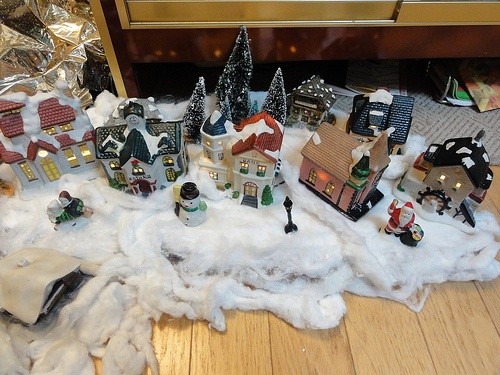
[384,198,417,237]
